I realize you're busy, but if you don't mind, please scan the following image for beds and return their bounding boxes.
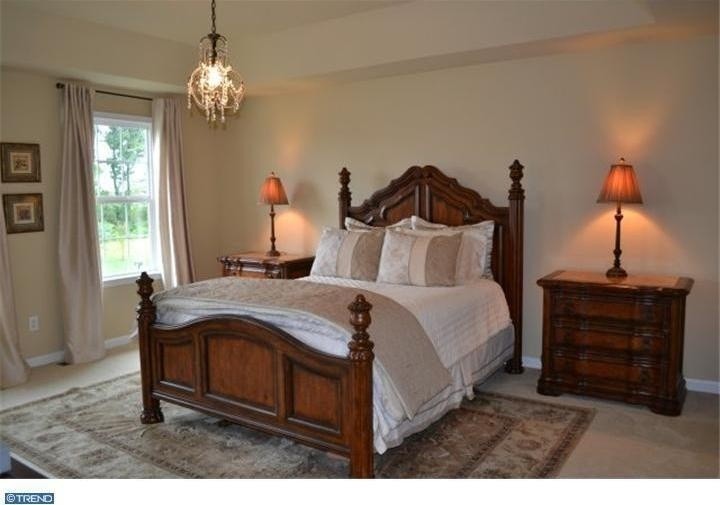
[136,160,525,479]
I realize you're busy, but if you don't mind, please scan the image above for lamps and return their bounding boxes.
[186,0,245,126]
[595,157,644,279]
[255,172,289,258]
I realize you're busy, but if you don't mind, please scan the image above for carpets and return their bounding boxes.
[0,368,601,479]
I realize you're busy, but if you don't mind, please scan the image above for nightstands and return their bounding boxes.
[217,250,315,281]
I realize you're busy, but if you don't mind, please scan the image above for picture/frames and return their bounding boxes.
[0,141,43,185]
[2,191,46,235]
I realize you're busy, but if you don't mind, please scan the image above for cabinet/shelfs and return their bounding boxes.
[536,270,695,417]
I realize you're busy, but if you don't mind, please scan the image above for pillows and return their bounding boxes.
[308,214,495,287]
